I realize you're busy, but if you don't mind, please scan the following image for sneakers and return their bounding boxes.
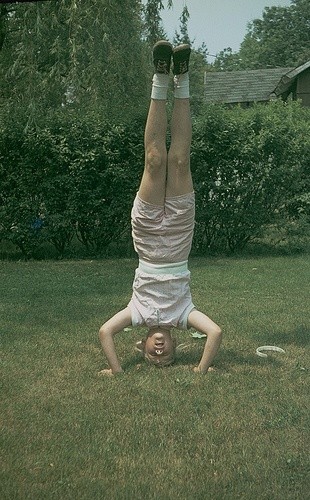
[151,40,173,75]
[173,44,191,76]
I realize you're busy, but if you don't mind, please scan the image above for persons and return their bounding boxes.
[99,40,227,374]
